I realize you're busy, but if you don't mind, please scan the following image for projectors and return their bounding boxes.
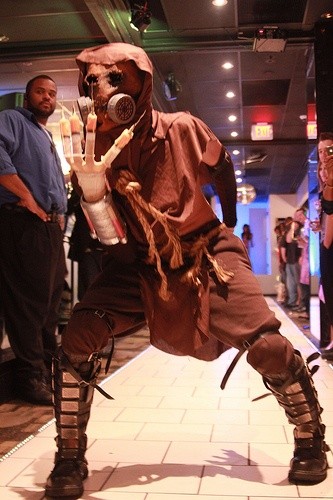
[252,29,288,53]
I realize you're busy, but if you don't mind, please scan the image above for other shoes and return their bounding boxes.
[291,304,306,312]
[284,303,298,307]
[20,377,59,403]
[320,346,333,361]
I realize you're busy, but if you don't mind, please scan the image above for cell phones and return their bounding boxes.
[326,145,333,156]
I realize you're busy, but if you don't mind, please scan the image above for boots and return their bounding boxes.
[39,355,102,500]
[262,349,329,485]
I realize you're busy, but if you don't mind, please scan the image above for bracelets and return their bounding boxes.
[320,196,333,215]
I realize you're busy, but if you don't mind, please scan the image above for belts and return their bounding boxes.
[5,203,59,222]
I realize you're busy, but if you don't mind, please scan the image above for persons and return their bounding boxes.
[45,42,329,498]
[65,170,111,302]
[1,75,67,406]
[238,130,333,365]
